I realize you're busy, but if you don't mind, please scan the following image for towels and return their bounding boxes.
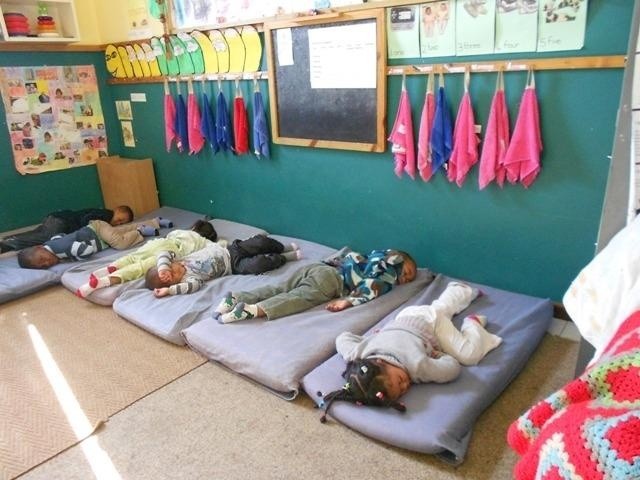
[448,67,480,184]
[234,78,249,156]
[188,76,200,155]
[429,69,452,180]
[475,66,510,189]
[416,70,437,182]
[387,73,416,179]
[504,68,542,185]
[252,75,271,161]
[217,77,233,155]
[201,80,216,153]
[174,79,188,154]
[164,80,175,154]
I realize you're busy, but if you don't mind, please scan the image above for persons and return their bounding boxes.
[18,217,173,270]
[1,205,134,254]
[76,220,218,299]
[214,249,416,324]
[145,235,301,299]
[314,283,503,425]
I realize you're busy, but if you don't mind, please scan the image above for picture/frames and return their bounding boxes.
[115,100,133,120]
[120,120,136,148]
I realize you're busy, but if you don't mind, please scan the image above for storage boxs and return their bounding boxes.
[0,1,82,43]
[97,157,161,220]
